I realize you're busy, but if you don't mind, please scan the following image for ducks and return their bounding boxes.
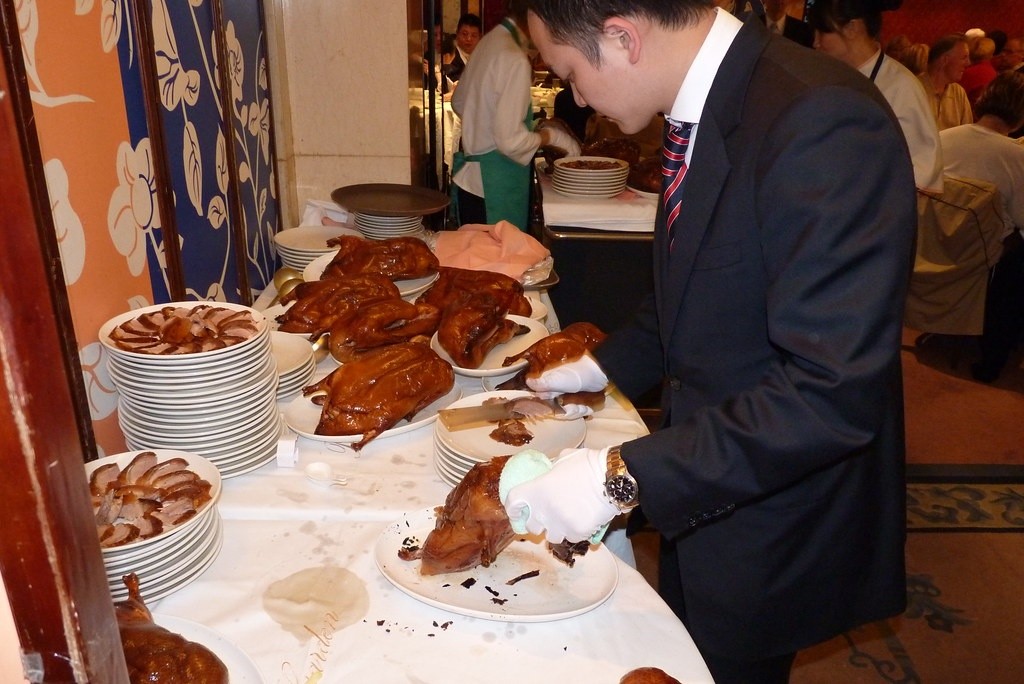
[274,274,438,363]
[422,265,531,369]
[300,338,454,451]
[318,233,440,279]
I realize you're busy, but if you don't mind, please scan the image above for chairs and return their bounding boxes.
[916,174,1024,384]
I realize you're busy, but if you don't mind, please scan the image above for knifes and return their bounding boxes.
[437,387,605,428]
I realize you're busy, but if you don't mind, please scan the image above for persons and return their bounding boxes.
[506,0,919,684]
[713,0,1024,377]
[433,0,669,306]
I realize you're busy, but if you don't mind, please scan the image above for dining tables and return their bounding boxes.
[145,268,713,684]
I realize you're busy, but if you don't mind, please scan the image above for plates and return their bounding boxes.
[83,449,222,605]
[482,370,524,391]
[273,225,364,275]
[551,156,628,199]
[303,251,439,296]
[626,185,658,199]
[149,612,263,684]
[433,391,588,490]
[94,301,287,482]
[537,161,553,178]
[271,330,315,401]
[329,182,450,241]
[279,382,463,443]
[521,296,547,320]
[430,314,549,377]
[376,505,618,623]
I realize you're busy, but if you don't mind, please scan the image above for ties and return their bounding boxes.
[660,122,694,258]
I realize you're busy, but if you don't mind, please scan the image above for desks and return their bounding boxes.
[545,188,660,242]
[443,102,552,187]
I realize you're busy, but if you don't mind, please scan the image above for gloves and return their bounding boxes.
[525,349,609,421]
[542,127,581,158]
[505,446,633,544]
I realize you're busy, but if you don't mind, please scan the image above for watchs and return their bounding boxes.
[605,444,639,508]
